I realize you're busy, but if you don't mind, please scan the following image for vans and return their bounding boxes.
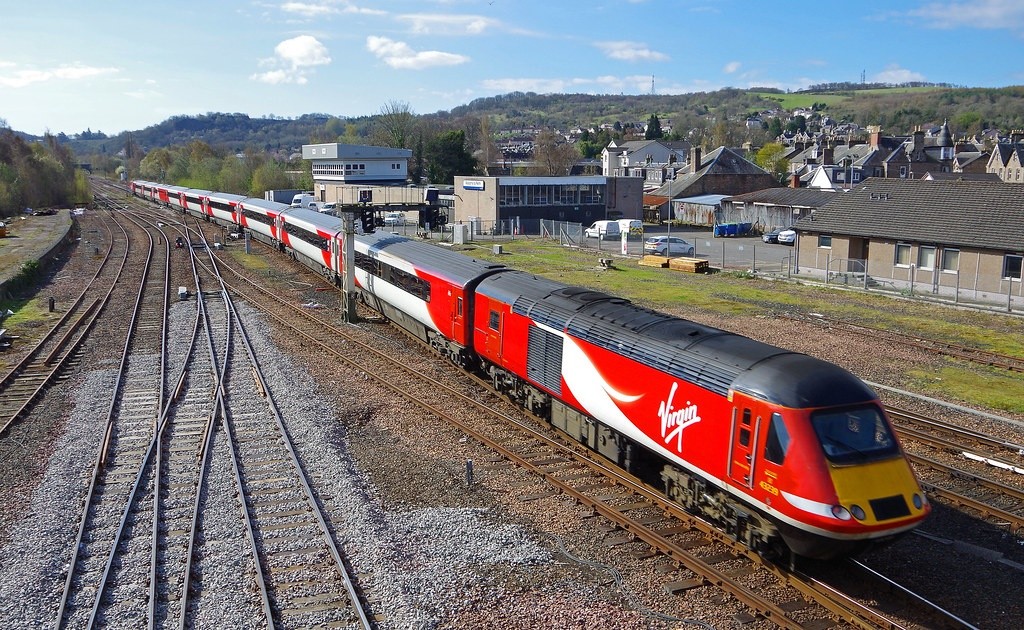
[291,193,314,208]
[308,201,338,215]
[617,219,644,237]
[584,220,621,240]
[385,212,407,226]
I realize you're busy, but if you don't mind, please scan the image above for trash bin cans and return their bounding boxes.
[0,226,6,237]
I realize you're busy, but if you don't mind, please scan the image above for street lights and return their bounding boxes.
[666,179,675,259]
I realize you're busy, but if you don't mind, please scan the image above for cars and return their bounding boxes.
[761,228,782,244]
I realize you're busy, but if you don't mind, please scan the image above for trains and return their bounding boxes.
[127,179,932,571]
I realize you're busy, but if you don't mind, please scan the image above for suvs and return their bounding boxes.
[644,235,695,255]
[777,226,796,246]
